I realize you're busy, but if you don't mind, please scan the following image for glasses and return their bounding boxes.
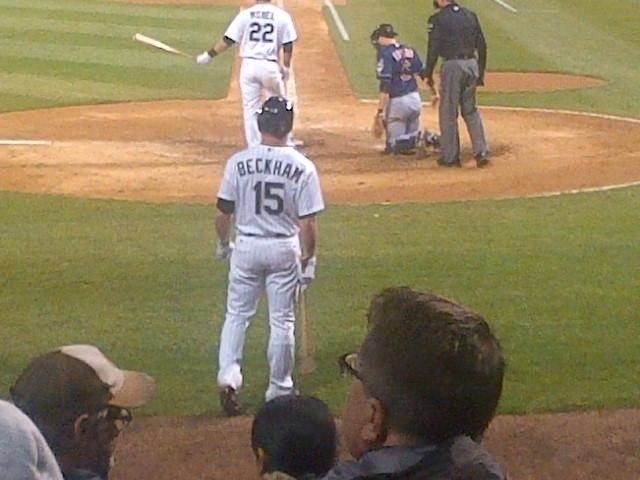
[112,408,131,431]
[336,351,362,381]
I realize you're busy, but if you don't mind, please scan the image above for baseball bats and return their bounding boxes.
[133,33,196,59]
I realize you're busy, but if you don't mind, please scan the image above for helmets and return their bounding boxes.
[370,24,399,41]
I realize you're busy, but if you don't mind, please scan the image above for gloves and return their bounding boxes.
[218,240,235,259]
[300,256,316,284]
[195,50,210,64]
[279,66,289,81]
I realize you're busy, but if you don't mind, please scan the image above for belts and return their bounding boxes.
[445,53,476,62]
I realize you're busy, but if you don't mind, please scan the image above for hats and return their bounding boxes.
[256,97,293,124]
[11,343,156,420]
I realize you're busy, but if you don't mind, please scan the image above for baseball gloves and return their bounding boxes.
[374,116,386,137]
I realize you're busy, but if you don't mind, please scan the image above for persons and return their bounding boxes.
[424,1,491,169]
[212,98,325,415]
[371,24,440,161]
[196,0,304,148]
[252,394,337,480]
[12,344,155,480]
[324,284,514,480]
[1,400,63,480]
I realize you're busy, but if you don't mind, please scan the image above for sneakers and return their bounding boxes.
[437,157,460,166]
[218,385,244,413]
[477,159,487,166]
